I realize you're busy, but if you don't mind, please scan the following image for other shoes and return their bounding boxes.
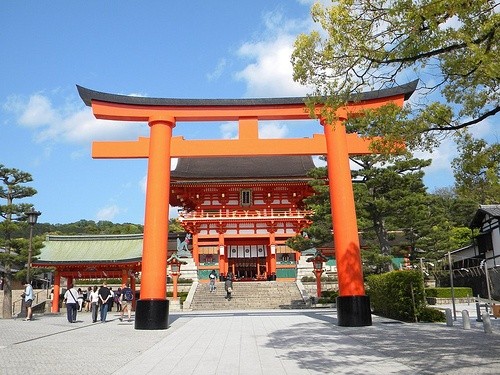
[119,318,123,321]
[128,318,130,322]
[69,320,72,323]
[73,320,76,323]
[23,317,30,321]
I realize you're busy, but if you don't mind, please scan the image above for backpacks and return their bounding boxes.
[125,289,132,300]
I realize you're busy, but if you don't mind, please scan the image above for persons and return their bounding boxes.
[209,269,217,292]
[89,286,99,323]
[21,279,34,320]
[119,283,134,321]
[50,288,122,312]
[64,284,78,323]
[99,281,111,321]
[225,277,232,300]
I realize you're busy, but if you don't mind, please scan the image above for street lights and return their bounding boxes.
[24,208,41,284]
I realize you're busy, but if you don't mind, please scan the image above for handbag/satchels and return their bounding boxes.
[76,301,80,310]
[228,287,232,293]
[210,274,214,279]
